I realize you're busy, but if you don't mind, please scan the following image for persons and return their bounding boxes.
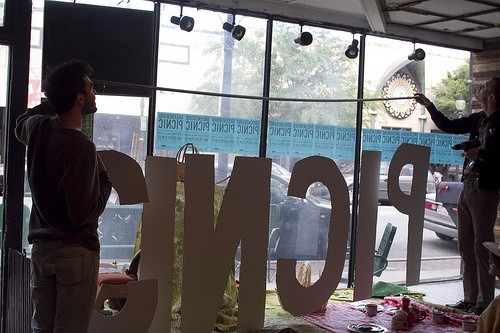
[14,61,113,333]
[414,76,500,316]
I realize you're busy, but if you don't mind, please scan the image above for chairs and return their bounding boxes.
[234,205,282,283]
[319,223,397,288]
[95,249,141,311]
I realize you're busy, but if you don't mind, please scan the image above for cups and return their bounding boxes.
[365,304,377,316]
[432,311,445,324]
[462,320,476,331]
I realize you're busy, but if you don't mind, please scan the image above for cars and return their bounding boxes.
[0,144,462,265]
[423,181,463,242]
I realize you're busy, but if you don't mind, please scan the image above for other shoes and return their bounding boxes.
[445,300,486,316]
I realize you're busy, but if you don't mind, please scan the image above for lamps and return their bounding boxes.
[294,20,313,46]
[408,38,425,61]
[345,29,359,58]
[223,10,246,41]
[171,1,194,32]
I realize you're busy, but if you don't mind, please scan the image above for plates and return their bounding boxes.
[350,304,384,312]
[357,324,383,332]
[346,323,388,333]
[387,307,401,314]
